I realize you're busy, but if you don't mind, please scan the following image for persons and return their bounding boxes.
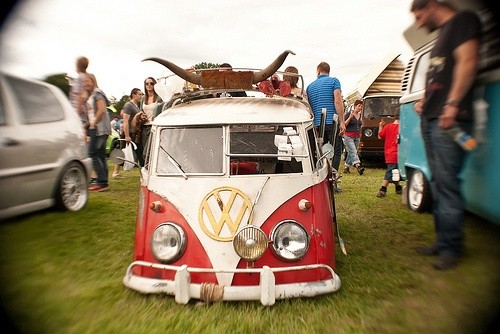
[376,108,403,198]
[213,63,247,97]
[138,77,163,168]
[307,62,346,192]
[70,57,98,185]
[283,66,308,100]
[341,99,365,175]
[110,116,120,130]
[112,88,147,179]
[115,107,124,167]
[82,73,112,192]
[407,0,483,268]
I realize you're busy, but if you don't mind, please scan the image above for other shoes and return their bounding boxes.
[416,245,439,257]
[376,186,387,197]
[357,166,365,175]
[396,185,403,193]
[88,185,109,191]
[434,257,457,270]
[344,168,351,174]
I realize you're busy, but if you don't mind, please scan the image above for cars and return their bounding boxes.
[0,70,96,223]
[119,48,346,308]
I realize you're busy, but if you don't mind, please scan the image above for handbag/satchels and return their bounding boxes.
[121,142,135,171]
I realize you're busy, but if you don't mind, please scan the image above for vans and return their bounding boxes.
[358,92,401,165]
[395,1,500,235]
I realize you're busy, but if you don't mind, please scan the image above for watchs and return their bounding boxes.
[444,99,462,107]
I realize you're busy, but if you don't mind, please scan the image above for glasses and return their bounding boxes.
[145,82,154,85]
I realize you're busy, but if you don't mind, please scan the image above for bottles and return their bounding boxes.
[447,125,477,152]
